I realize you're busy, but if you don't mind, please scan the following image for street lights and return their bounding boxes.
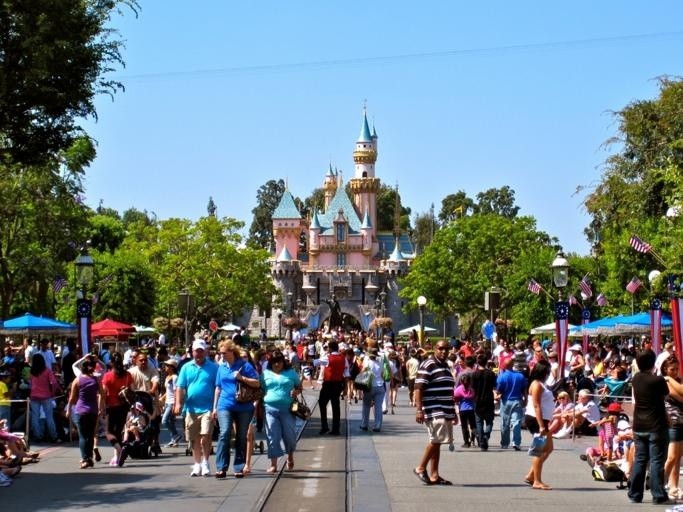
[550,249,570,302]
[416,295,428,348]
[379,291,387,340]
[503,297,510,336]
[296,298,302,318]
[578,287,593,311]
[73,245,95,299]
[176,288,196,349]
[375,297,380,340]
[276,308,283,340]
[646,269,664,298]
[285,289,294,341]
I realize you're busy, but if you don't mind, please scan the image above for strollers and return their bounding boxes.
[230,420,264,455]
[599,374,635,425]
[185,436,214,456]
[118,390,160,458]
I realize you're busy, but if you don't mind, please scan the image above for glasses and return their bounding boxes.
[138,349,149,354]
[559,397,564,399]
[436,346,448,350]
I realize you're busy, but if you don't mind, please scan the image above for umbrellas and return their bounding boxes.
[0,312,77,331]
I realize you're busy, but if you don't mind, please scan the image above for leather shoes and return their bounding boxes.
[235,470,243,478]
[21,452,39,464]
[328,430,340,435]
[8,465,21,477]
[50,437,63,444]
[215,470,226,478]
[319,428,330,435]
[449,445,454,451]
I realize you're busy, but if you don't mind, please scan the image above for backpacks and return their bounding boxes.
[117,372,135,408]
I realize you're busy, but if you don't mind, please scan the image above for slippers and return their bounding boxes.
[523,478,552,490]
[412,468,431,484]
[428,475,452,485]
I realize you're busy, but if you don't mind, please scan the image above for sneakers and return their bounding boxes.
[551,429,571,439]
[0,471,13,487]
[652,487,683,505]
[359,426,368,432]
[512,440,520,450]
[190,457,211,476]
[109,446,128,468]
[502,444,508,449]
[372,429,380,432]
[627,490,644,502]
[476,434,488,450]
[79,457,94,469]
[461,440,476,448]
[98,432,106,438]
[93,448,101,462]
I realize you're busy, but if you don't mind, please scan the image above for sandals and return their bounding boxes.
[266,466,277,473]
[286,457,294,470]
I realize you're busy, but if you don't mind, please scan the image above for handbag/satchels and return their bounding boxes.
[592,460,628,482]
[349,363,360,377]
[235,361,266,403]
[354,359,374,390]
[382,355,391,382]
[289,386,312,421]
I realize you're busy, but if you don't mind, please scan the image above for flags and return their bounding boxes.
[567,296,578,306]
[626,235,651,255]
[594,292,605,307]
[624,274,641,296]
[526,278,542,296]
[575,274,594,298]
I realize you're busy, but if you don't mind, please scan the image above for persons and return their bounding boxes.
[624,347,679,504]
[0,316,682,491]
[656,353,682,500]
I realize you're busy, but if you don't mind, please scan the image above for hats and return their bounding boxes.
[608,403,624,412]
[548,352,558,357]
[365,340,379,358]
[617,419,631,430]
[510,351,526,361]
[130,401,144,412]
[391,351,398,358]
[164,359,178,368]
[192,338,207,351]
[569,344,582,351]
